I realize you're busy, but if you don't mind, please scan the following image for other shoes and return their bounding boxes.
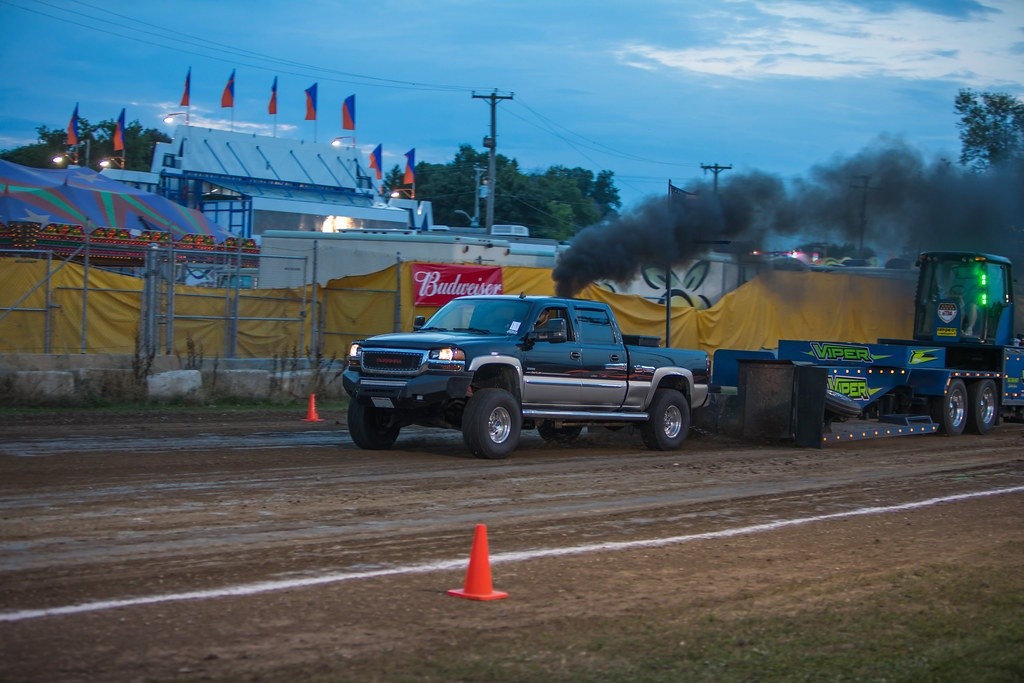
[964,330,972,336]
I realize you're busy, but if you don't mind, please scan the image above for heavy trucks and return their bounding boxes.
[691,249,1024,451]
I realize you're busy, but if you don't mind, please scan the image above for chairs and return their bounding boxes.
[542,318,569,340]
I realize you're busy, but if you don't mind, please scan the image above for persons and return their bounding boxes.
[535,309,553,338]
[959,303,978,336]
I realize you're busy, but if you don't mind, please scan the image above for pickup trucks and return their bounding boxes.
[340,292,712,464]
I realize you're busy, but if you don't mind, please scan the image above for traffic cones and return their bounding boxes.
[301,393,325,422]
[447,523,509,603]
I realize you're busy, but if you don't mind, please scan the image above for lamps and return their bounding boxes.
[330,135,357,148]
[54,145,79,166]
[389,188,416,201]
[163,112,189,125]
[98,155,126,170]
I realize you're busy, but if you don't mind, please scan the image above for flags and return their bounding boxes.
[181,71,190,106]
[403,148,414,185]
[370,144,381,179]
[343,95,354,130]
[113,108,124,151]
[222,72,234,107]
[66,106,78,145]
[269,80,276,114]
[305,83,317,120]
